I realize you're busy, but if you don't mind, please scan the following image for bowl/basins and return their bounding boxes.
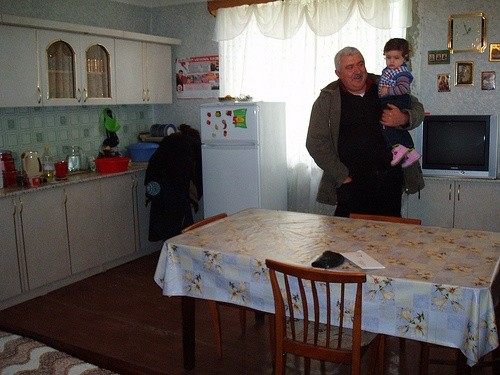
[124,142,160,162]
[0,150,18,186]
[94,157,129,174]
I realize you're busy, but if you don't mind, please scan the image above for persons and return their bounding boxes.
[377,38,420,169]
[176,70,183,90]
[439,76,446,90]
[459,64,471,84]
[307,46,426,218]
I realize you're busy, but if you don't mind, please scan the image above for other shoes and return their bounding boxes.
[402,150,420,168]
[390,145,409,166]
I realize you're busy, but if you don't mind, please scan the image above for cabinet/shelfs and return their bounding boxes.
[0,171,204,313]
[0,16,182,107]
[402,176,500,233]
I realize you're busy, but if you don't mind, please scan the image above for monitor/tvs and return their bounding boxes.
[414,112,497,179]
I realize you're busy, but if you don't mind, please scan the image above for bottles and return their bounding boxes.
[42,145,55,182]
[16,171,27,189]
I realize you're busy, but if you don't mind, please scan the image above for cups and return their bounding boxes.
[54,161,67,181]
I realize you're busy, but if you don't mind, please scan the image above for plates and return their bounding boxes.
[149,123,177,137]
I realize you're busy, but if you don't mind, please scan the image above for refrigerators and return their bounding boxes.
[199,100,288,219]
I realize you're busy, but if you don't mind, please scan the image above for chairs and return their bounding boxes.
[182,214,500,375]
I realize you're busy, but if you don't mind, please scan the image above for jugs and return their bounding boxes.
[21,150,42,182]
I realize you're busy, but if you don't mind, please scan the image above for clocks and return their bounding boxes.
[446,11,487,54]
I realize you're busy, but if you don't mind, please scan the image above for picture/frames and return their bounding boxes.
[454,61,474,86]
[436,73,451,92]
[481,71,496,90]
[427,50,450,64]
[489,43,500,62]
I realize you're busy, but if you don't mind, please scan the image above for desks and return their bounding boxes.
[154,207,500,375]
